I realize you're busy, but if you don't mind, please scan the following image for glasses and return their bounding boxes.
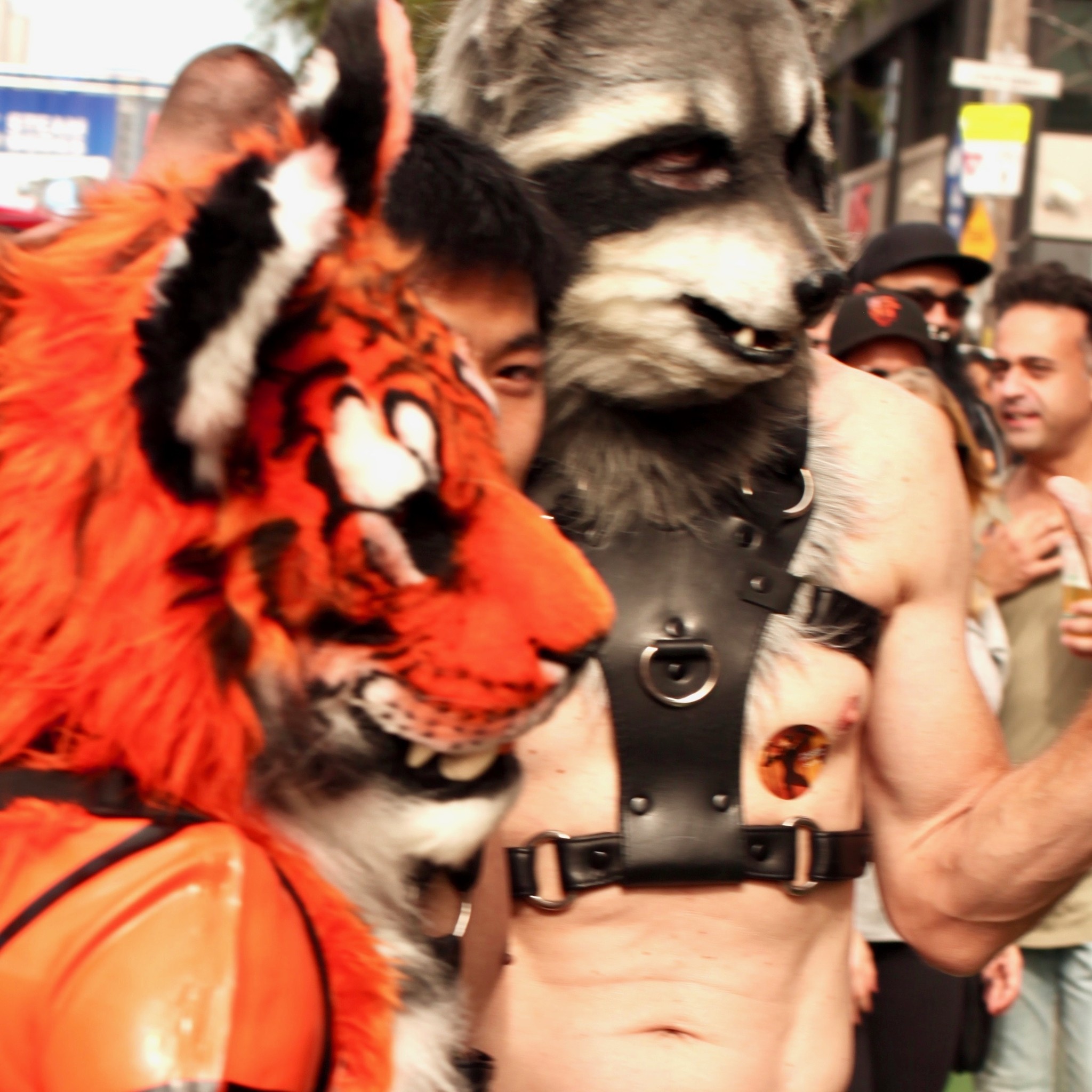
[860,364,890,379]
[875,286,973,320]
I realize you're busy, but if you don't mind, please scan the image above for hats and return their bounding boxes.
[854,222,992,287]
[829,291,938,366]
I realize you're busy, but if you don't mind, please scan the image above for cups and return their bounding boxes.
[1058,533,1092,615]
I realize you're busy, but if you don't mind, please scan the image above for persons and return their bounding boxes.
[0,111,550,1092]
[802,226,1092,1092]
[418,0,1092,1092]
[130,45,299,189]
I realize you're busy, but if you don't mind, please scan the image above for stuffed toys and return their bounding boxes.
[0,157,612,1092]
